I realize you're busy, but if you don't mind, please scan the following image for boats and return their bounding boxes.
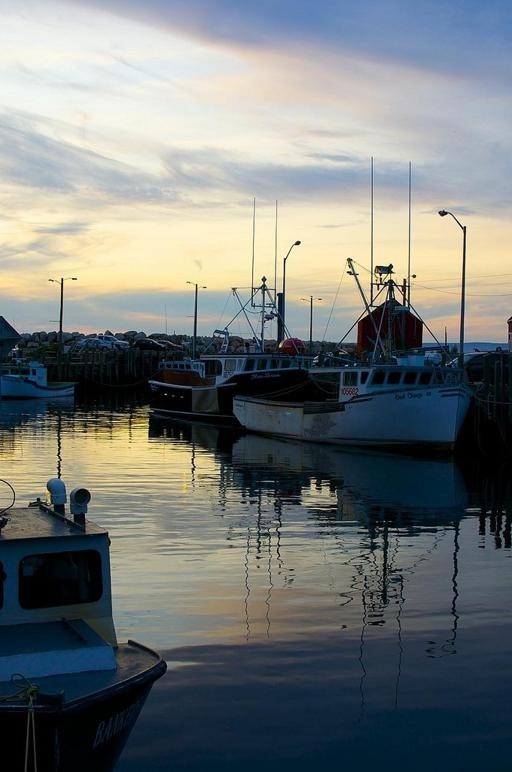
[0,356,81,398]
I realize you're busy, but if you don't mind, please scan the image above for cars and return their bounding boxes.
[74,335,129,354]
[133,338,184,351]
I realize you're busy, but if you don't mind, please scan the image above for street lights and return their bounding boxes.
[185,281,208,360]
[48,277,78,333]
[437,209,468,367]
[281,240,302,344]
[300,295,322,342]
